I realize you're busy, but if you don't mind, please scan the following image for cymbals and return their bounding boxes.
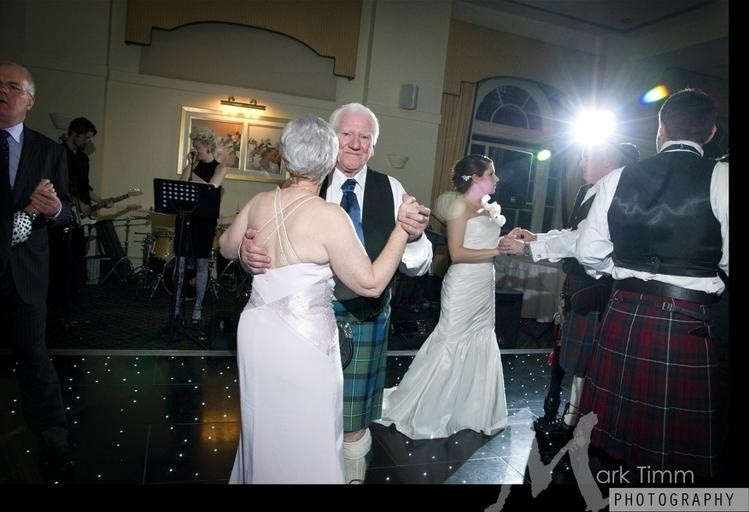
[136,208,170,217]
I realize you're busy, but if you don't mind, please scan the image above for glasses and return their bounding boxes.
[0,82,35,101]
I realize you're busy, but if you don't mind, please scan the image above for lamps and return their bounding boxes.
[218,95,267,120]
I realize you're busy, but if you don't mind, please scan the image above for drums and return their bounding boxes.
[147,229,176,261]
[161,255,210,300]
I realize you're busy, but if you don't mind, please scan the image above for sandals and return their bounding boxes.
[162,309,203,325]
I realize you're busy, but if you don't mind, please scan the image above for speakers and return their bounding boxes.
[399,83,419,109]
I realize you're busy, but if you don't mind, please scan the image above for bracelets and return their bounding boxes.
[237,239,244,262]
[29,212,38,222]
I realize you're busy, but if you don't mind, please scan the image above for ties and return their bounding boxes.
[0,130,9,179]
[340,178,365,246]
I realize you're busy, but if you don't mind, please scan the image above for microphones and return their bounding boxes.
[191,149,197,155]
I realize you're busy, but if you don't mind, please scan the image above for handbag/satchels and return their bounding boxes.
[552,293,570,350]
[337,321,353,369]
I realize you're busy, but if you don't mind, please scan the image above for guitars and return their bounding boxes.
[74,187,144,228]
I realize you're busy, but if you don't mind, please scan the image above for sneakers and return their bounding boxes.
[545,394,560,414]
[534,418,576,437]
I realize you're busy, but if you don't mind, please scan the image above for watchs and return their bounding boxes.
[524,241,531,256]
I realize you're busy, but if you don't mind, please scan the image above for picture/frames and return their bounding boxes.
[176,105,295,184]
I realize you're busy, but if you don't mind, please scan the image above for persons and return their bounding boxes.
[437,154,527,436]
[0,172,58,272]
[571,89,730,489]
[495,135,626,438]
[0,60,92,486]
[217,132,280,172]
[168,126,228,326]
[57,117,114,328]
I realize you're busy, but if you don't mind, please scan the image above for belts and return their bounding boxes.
[613,277,721,307]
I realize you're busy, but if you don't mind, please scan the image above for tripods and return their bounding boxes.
[206,255,248,304]
[96,224,136,289]
[141,214,209,350]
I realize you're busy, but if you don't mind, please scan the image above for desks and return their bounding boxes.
[495,251,569,350]
